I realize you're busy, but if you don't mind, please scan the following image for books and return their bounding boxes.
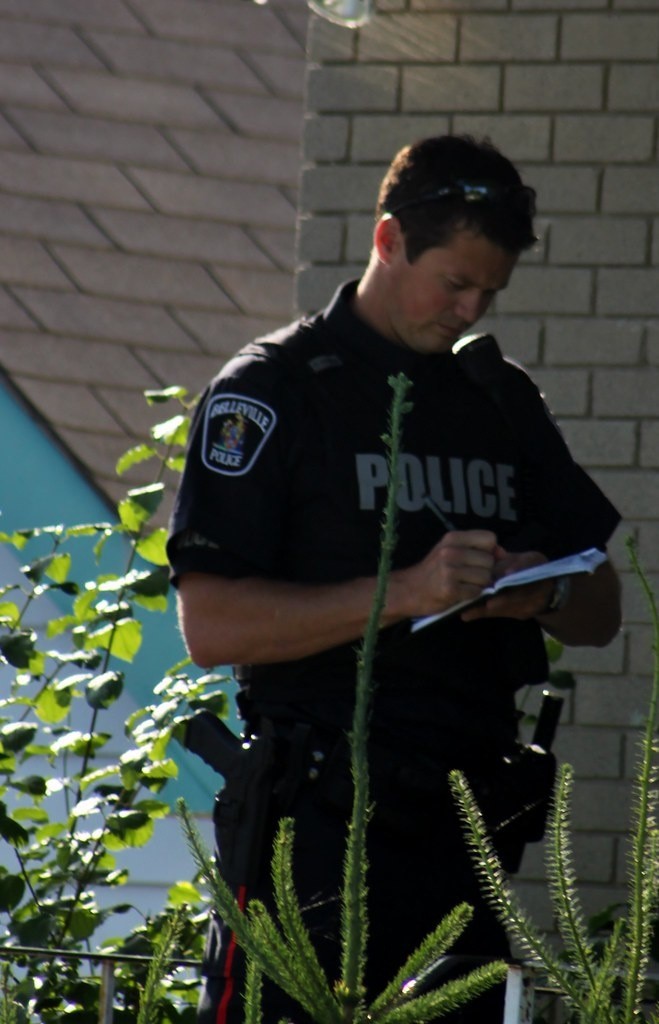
[411,547,607,634]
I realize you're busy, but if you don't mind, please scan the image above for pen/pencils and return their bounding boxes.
[420,494,458,532]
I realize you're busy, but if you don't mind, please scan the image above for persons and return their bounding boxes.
[164,136,623,1023]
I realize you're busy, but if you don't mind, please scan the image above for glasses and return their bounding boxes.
[391,169,537,219]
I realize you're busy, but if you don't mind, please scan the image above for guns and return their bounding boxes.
[168,700,294,891]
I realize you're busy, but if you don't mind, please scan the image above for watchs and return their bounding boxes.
[542,578,570,614]
